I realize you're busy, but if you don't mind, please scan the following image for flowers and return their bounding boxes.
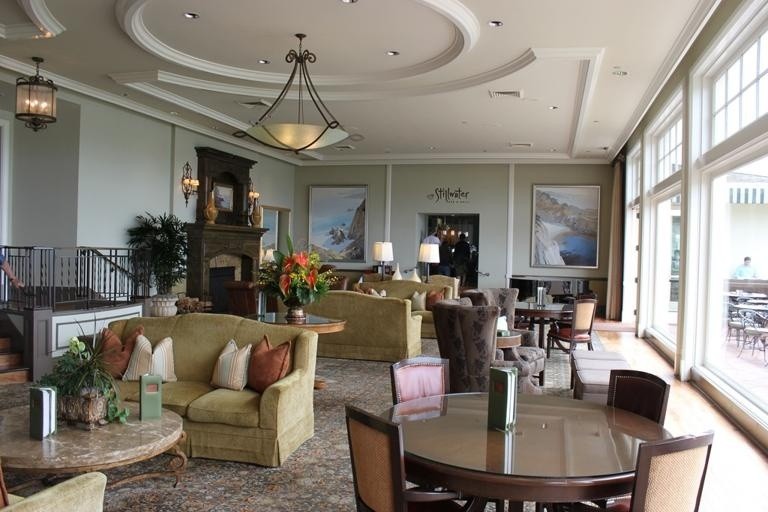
[249,233,334,306]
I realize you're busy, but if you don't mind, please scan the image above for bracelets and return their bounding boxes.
[9,277,17,281]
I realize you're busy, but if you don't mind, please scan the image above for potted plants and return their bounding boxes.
[124,208,192,317]
[25,309,132,431]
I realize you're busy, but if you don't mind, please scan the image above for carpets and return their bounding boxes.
[6,327,641,512]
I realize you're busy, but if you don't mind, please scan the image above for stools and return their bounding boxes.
[565,348,637,402]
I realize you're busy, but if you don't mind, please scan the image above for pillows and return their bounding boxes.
[121,334,179,385]
[100,323,145,381]
[207,337,251,395]
[246,332,292,396]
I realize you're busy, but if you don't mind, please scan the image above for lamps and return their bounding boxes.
[372,241,396,282]
[246,177,259,214]
[416,240,440,283]
[232,32,352,156]
[11,54,59,130]
[179,159,199,208]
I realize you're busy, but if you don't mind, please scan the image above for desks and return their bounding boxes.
[490,321,523,354]
[244,311,348,391]
[0,400,187,496]
[373,396,671,512]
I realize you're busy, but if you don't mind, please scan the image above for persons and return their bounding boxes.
[732,256,758,280]
[431,233,478,287]
[0,254,24,289]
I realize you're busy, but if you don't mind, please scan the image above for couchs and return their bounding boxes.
[0,461,107,512]
[303,272,463,366]
[101,312,319,469]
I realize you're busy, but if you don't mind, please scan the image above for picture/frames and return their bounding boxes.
[211,182,236,214]
[526,180,602,270]
[305,183,370,265]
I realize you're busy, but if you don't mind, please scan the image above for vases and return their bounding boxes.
[283,316,307,326]
[202,191,220,226]
[285,304,307,319]
[248,197,262,228]
[390,262,401,281]
[407,267,421,283]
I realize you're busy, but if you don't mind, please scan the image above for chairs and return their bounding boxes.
[431,297,536,396]
[534,368,673,512]
[388,394,449,425]
[457,284,547,387]
[341,401,465,512]
[221,280,266,323]
[513,290,599,355]
[603,403,664,443]
[386,354,505,511]
[587,427,716,512]
[726,287,768,363]
